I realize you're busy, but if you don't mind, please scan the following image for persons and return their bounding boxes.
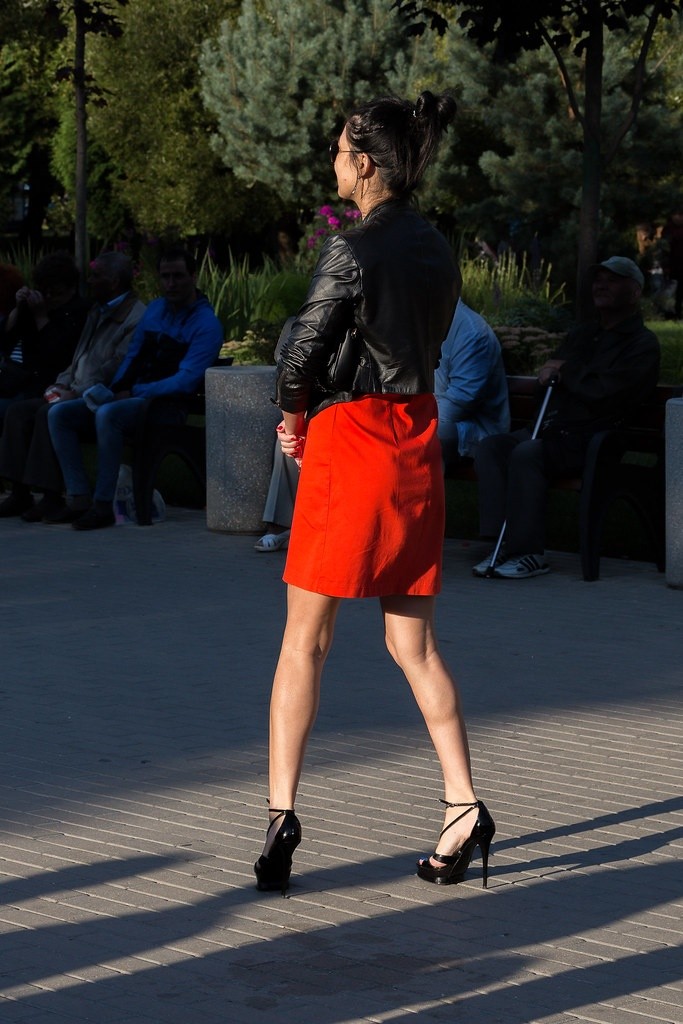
[472,257,661,580]
[433,297,511,480]
[253,439,298,551]
[0,254,89,419]
[42,249,223,528]
[254,90,496,889]
[0,252,147,523]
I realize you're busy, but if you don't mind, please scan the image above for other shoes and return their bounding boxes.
[21,497,63,523]
[0,495,35,518]
[41,502,90,524]
[72,507,116,530]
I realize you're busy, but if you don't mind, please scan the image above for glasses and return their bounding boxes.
[329,139,374,163]
[42,288,69,298]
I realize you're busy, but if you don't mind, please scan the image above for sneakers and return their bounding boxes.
[493,553,551,578]
[473,547,515,578]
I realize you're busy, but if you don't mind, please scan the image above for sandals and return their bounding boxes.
[253,529,291,551]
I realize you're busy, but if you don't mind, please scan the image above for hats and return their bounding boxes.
[586,256,644,289]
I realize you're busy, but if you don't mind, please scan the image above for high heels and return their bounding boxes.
[254,798,302,898]
[416,799,496,889]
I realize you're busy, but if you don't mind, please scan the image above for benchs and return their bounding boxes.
[439,373,683,585]
